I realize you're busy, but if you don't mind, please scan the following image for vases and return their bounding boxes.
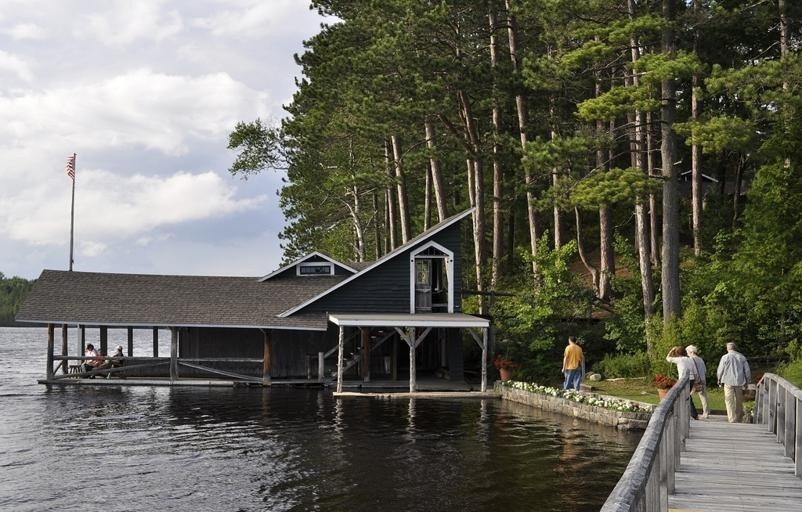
[657,388,670,401]
[498,368,513,381]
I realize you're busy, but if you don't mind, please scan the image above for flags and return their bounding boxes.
[66,151,77,181]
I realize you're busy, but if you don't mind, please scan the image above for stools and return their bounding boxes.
[68,364,83,380]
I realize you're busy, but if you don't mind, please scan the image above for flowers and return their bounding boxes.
[655,375,677,390]
[493,353,521,369]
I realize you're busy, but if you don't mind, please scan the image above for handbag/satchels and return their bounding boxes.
[694,381,704,391]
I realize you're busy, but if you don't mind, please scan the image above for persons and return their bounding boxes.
[106,344,124,366]
[716,341,752,423]
[666,344,700,422]
[80,342,101,375]
[686,342,710,420]
[561,336,584,392]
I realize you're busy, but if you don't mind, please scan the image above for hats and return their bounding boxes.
[686,345,697,353]
[117,345,122,350]
